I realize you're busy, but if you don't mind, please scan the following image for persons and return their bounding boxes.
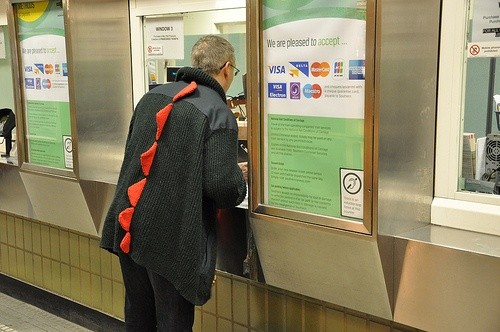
[100,33,248,332]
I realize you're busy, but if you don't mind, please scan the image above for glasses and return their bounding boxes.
[220,63,240,75]
